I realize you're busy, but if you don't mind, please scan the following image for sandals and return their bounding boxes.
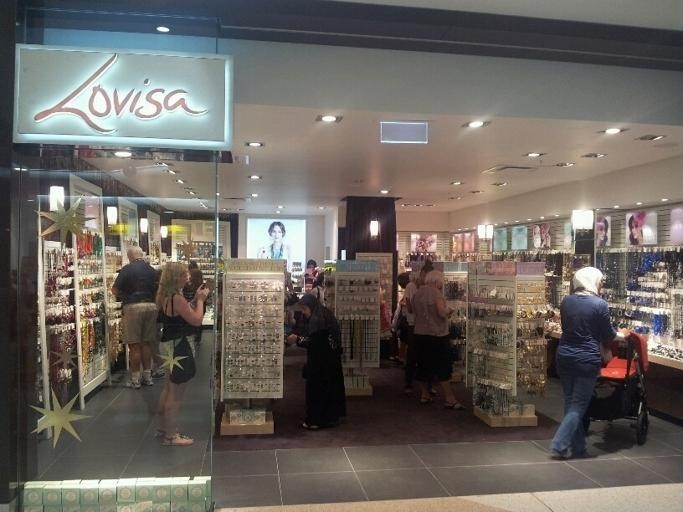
[405,387,466,410]
[155,427,195,446]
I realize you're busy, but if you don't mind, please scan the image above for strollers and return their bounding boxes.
[582,328,651,445]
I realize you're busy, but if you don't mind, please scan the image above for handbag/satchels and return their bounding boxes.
[169,336,196,384]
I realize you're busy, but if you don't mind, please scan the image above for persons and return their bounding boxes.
[188,261,207,345]
[533,224,541,248]
[380,271,410,369]
[153,260,211,450]
[410,269,466,411]
[111,245,161,390]
[597,216,609,247]
[257,220,296,260]
[306,259,318,269]
[394,260,438,396]
[547,266,629,461]
[309,271,327,309]
[286,293,348,431]
[541,222,551,247]
[628,215,646,245]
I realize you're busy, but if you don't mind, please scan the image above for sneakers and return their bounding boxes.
[122,368,164,389]
[299,422,317,432]
[571,449,601,458]
